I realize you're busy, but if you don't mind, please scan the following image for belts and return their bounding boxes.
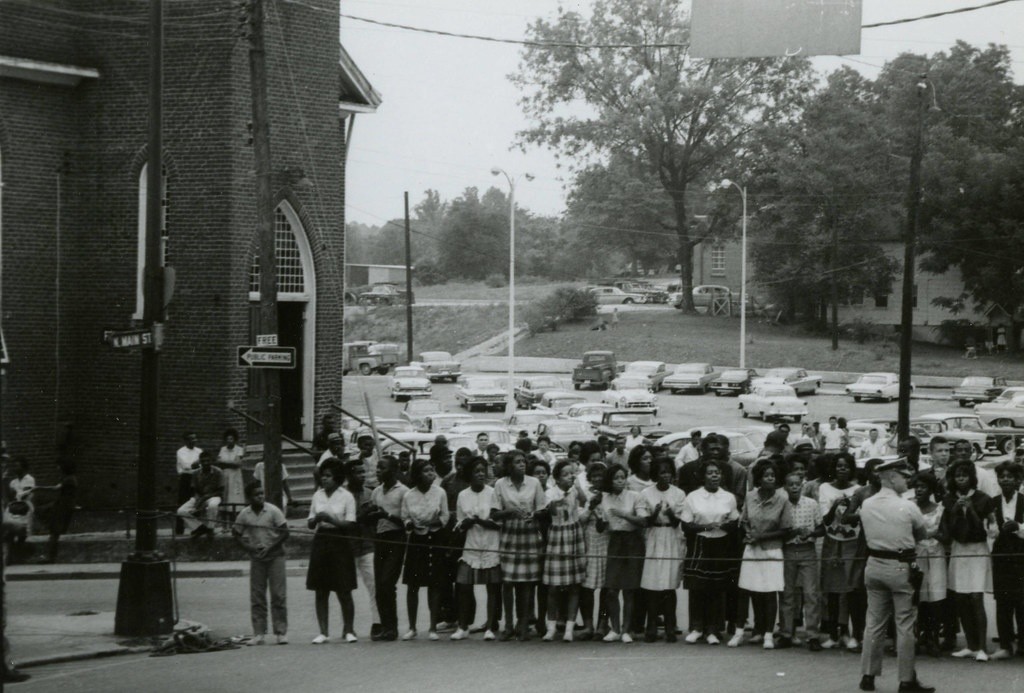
[868,547,916,560]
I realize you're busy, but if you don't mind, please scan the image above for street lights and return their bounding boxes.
[491,167,535,425]
[721,179,747,368]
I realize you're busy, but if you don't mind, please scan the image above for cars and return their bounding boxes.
[748,366,823,396]
[357,284,415,306]
[952,376,1009,407]
[738,386,810,422]
[456,378,509,412]
[388,366,433,402]
[708,369,759,396]
[342,386,1024,466]
[409,351,462,383]
[513,376,565,410]
[611,361,673,393]
[845,372,915,403]
[578,278,740,310]
[662,362,721,395]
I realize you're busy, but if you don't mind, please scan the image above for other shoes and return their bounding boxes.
[808,638,821,651]
[859,674,877,690]
[791,636,803,645]
[992,632,1016,642]
[820,638,840,648]
[847,638,859,648]
[975,649,988,661]
[951,648,976,657]
[775,638,792,649]
[840,635,850,645]
[247,635,265,646]
[346,632,358,643]
[762,632,775,649]
[884,644,898,656]
[898,679,936,693]
[727,628,744,647]
[939,639,958,651]
[312,634,330,644]
[191,523,215,537]
[748,633,762,643]
[371,612,734,644]
[277,633,289,644]
[989,649,1012,661]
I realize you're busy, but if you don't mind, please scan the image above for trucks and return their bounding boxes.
[342,340,399,376]
[572,350,625,390]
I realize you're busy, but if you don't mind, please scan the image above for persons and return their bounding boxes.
[306,416,1024,663]
[175,431,204,534]
[592,321,608,330]
[217,429,245,511]
[0,438,84,566]
[611,308,618,330]
[985,325,994,355]
[252,453,293,516]
[176,451,224,538]
[997,323,1007,354]
[962,335,978,350]
[858,457,936,693]
[231,480,290,644]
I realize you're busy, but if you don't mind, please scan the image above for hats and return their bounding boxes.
[874,458,912,478]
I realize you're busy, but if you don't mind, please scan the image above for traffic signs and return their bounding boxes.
[236,344,296,369]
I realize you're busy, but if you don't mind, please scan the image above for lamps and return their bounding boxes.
[283,166,315,188]
